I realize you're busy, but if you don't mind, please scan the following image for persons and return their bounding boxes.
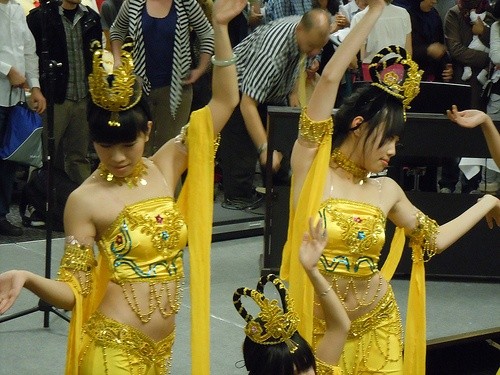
[0,0,248,375]
[260,0,368,78]
[1,0,47,239]
[406,1,457,82]
[230,215,354,374]
[463,1,492,86]
[110,0,217,200]
[485,0,499,135]
[442,1,491,113]
[281,0,500,375]
[348,0,413,85]
[446,104,500,171]
[220,9,334,208]
[20,0,107,235]
[99,0,126,50]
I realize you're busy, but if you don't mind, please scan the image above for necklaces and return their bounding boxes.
[329,147,373,184]
[96,156,150,191]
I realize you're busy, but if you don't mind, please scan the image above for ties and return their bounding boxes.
[298,54,307,108]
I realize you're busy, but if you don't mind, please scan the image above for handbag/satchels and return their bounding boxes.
[39,60,64,102]
[1,84,45,168]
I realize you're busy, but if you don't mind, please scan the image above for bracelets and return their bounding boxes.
[315,285,333,298]
[211,52,239,67]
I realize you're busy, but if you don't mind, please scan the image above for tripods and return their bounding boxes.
[0,0,74,329]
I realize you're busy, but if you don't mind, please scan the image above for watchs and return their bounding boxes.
[257,142,269,156]
